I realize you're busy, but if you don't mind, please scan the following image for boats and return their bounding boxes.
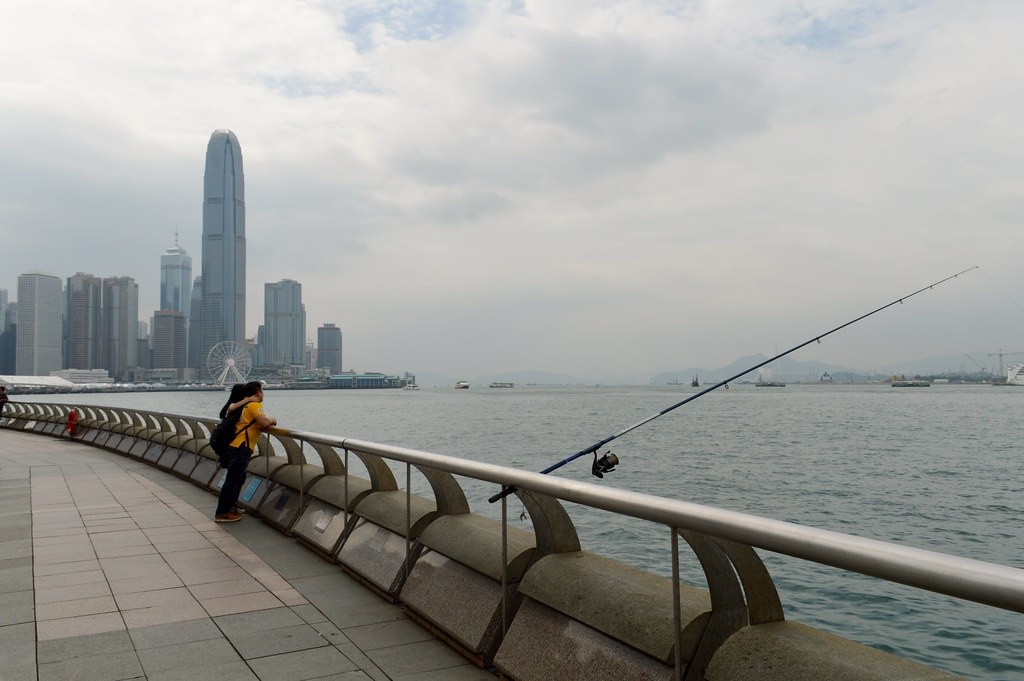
[404,382,420,389]
[666,373,716,386]
[755,373,785,387]
[454,380,469,389]
[489,383,514,388]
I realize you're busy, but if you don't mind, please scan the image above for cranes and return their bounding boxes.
[989,348,1024,376]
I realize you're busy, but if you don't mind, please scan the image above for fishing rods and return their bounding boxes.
[487,263,980,505]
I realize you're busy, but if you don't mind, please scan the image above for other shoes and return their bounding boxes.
[230,506,246,514]
[215,513,242,521]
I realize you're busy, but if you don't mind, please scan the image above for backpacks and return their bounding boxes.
[209,400,255,454]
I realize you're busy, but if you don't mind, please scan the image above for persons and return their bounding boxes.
[214,381,277,522]
[0,386,8,418]
